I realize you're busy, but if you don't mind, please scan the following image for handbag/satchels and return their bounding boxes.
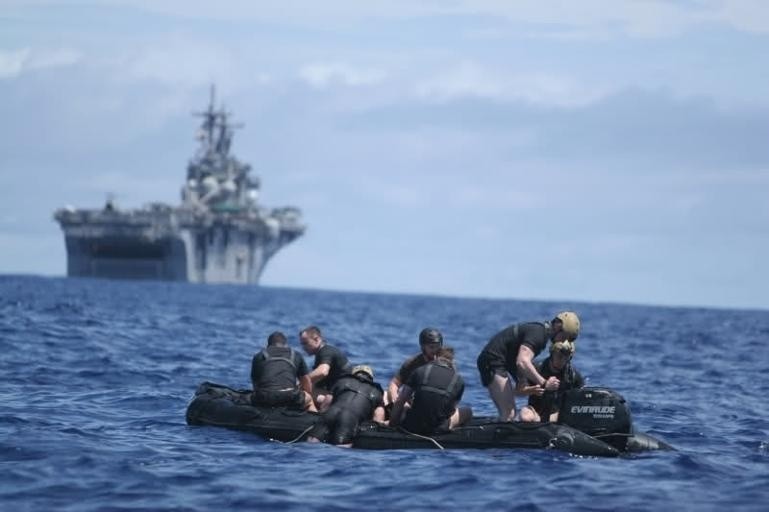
[555,383,635,434]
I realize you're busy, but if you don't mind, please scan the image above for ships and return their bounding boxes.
[54,84,306,284]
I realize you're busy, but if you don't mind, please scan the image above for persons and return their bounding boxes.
[389,347,474,435]
[477,311,580,422]
[298,326,353,412]
[247,332,319,415]
[307,365,386,448]
[387,327,443,404]
[519,339,586,421]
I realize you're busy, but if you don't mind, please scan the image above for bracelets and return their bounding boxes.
[541,379,548,388]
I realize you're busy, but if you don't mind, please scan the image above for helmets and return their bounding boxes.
[549,339,575,357]
[555,311,580,343]
[418,327,444,348]
[350,364,375,379]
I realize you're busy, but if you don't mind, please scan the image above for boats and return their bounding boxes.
[184,380,677,457]
